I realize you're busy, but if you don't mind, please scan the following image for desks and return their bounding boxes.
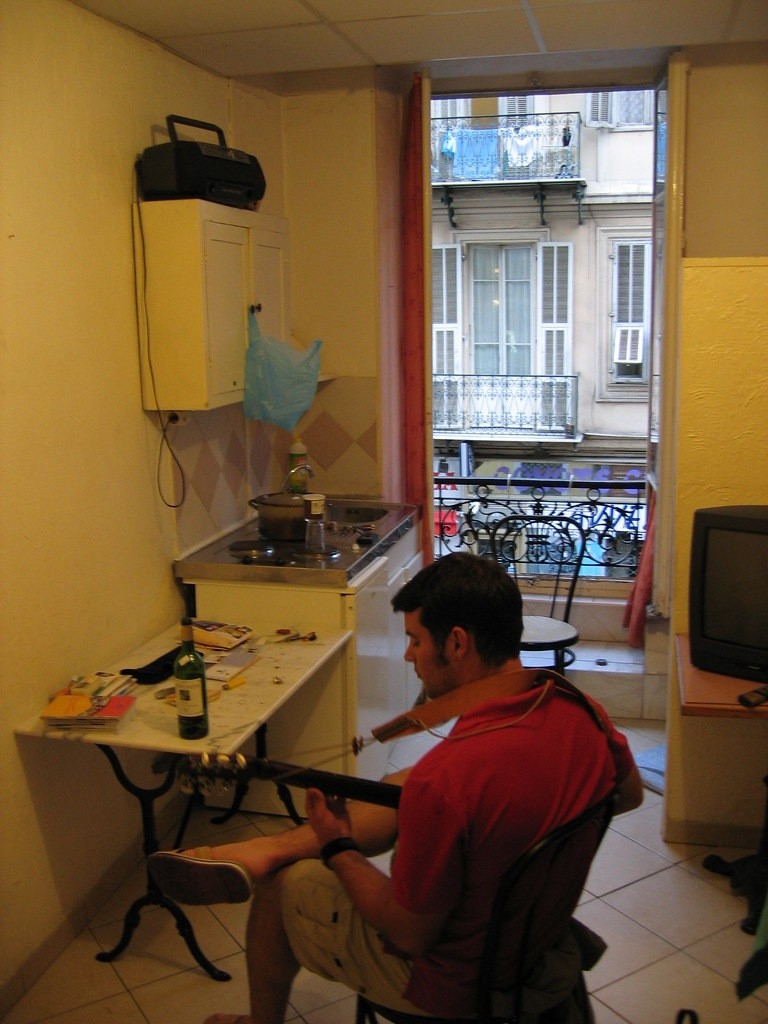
[13,617,353,981]
[674,633,768,936]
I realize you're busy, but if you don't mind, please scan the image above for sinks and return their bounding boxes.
[322,499,390,525]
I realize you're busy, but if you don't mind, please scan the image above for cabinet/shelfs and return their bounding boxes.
[130,199,293,413]
[183,519,426,818]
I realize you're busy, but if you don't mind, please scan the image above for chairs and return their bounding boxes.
[490,515,586,677]
[355,790,623,1024]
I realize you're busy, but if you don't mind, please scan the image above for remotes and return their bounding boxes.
[738,685,768,707]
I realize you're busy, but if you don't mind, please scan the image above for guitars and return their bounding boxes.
[174,752,403,810]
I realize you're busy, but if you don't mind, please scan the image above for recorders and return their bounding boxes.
[136,113,266,211]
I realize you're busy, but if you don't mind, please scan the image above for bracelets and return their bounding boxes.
[321,836,358,869]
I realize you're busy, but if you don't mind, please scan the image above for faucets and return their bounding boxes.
[279,464,314,493]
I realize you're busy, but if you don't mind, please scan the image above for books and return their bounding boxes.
[38,671,139,735]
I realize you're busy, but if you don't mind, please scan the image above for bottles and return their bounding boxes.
[173,617,209,740]
[289,438,308,492]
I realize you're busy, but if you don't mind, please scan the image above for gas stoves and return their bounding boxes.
[173,499,424,588]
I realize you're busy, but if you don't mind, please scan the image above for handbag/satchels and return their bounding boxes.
[244,309,322,432]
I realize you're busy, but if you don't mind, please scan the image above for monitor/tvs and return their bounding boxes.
[688,505,768,684]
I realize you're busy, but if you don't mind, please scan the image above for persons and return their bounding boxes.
[145,552,644,1024]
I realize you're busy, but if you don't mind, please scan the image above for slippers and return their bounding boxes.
[203,1013,250,1024]
[146,848,253,905]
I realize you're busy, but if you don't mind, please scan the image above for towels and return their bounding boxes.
[453,129,499,180]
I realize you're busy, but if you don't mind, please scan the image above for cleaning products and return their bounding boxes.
[288,434,309,493]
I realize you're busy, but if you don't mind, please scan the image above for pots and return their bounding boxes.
[248,492,313,542]
